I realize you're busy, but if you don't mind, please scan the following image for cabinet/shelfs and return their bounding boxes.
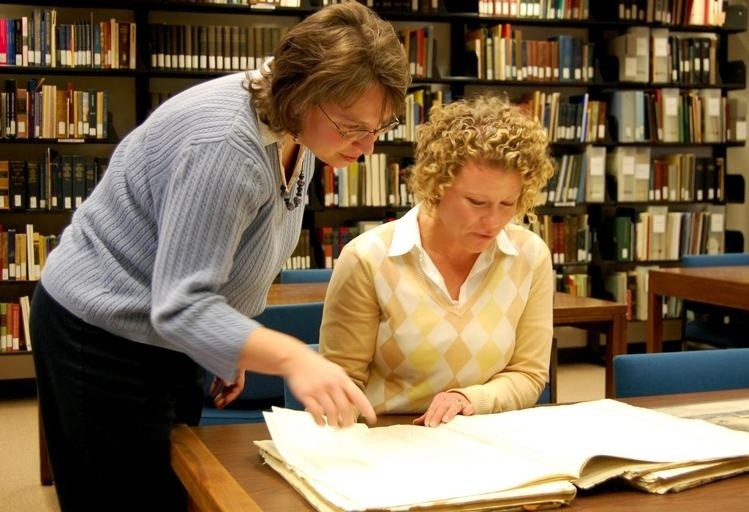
[0,0,749,382]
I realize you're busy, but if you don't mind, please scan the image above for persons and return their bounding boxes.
[30,0,413,512]
[303,96,554,426]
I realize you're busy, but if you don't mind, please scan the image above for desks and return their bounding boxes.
[162,390,749,512]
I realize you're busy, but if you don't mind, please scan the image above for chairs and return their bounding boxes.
[200,303,323,425]
[267,249,748,388]
[613,348,749,398]
[282,344,320,411]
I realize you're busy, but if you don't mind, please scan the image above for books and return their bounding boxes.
[324,0,452,145]
[462,0,747,323]
[253,398,749,512]
[281,230,311,270]
[150,91,175,113]
[0,6,136,352]
[322,152,415,270]
[148,23,281,72]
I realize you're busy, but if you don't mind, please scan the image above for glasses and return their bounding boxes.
[316,103,400,142]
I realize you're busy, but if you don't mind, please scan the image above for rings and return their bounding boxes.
[456,398,462,404]
